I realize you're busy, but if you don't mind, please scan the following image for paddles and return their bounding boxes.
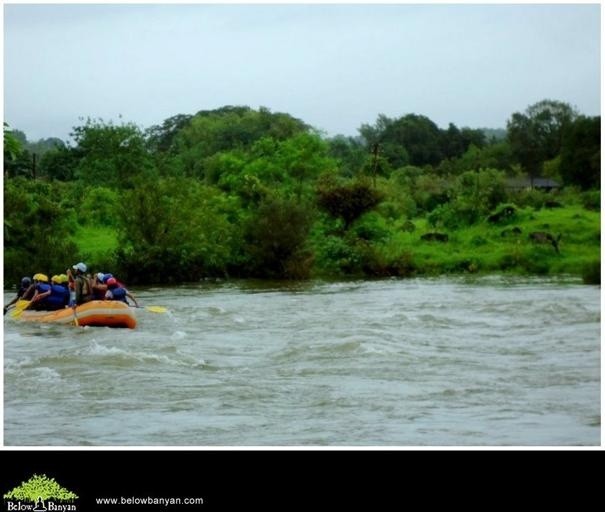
[129,306,167,312]
[72,309,79,327]
[4,300,32,320]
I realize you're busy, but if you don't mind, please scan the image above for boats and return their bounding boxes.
[17,300,136,329]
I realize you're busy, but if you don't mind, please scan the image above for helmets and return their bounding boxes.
[21,262,118,289]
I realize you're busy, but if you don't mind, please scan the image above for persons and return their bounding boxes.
[4,262,139,312]
